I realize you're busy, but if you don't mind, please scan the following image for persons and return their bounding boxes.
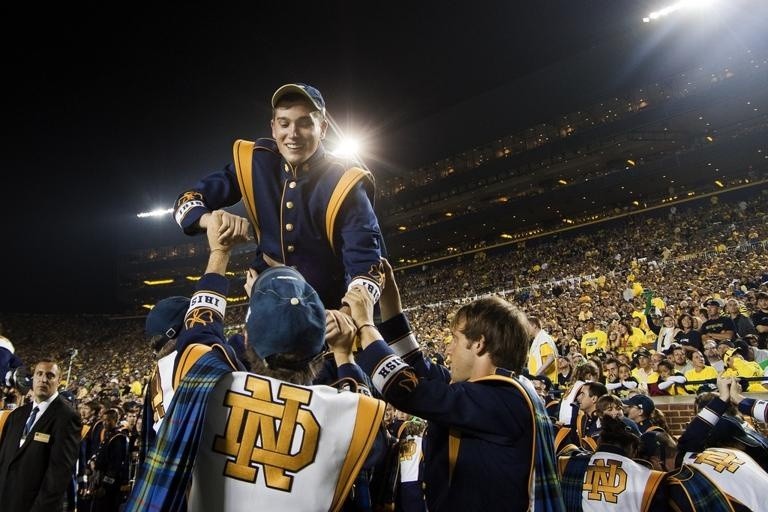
[558,361,599,427]
[530,375,553,407]
[684,350,718,394]
[617,354,629,364]
[78,401,105,512]
[526,316,558,390]
[633,429,768,512]
[667,346,697,374]
[558,357,573,388]
[174,83,386,337]
[633,347,659,383]
[728,299,759,341]
[0,392,21,438]
[428,260,458,301]
[459,257,495,297]
[605,363,639,398]
[751,291,768,349]
[128,209,389,510]
[675,370,768,472]
[580,319,607,358]
[408,303,473,365]
[146,295,191,436]
[88,410,130,511]
[224,306,247,341]
[606,329,620,357]
[87,320,155,403]
[721,347,768,392]
[657,315,683,354]
[554,205,768,290]
[656,359,690,395]
[121,409,137,427]
[670,314,703,351]
[621,394,658,433]
[56,357,84,400]
[549,416,668,511]
[0,312,87,356]
[575,382,611,452]
[652,351,669,383]
[733,340,768,376]
[711,339,734,373]
[596,394,641,436]
[0,356,84,512]
[557,291,579,355]
[2,357,31,392]
[700,300,734,348]
[616,321,643,362]
[130,415,143,479]
[498,257,552,314]
[341,256,553,512]
[631,351,641,376]
[583,288,699,314]
[606,358,622,383]
[396,266,427,310]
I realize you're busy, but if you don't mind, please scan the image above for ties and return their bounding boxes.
[20,407,39,448]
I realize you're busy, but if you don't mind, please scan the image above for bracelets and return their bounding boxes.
[358,323,379,332]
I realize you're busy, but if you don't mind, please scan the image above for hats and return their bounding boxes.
[246,266,325,371]
[704,300,720,307]
[146,296,190,353]
[640,430,679,458]
[723,347,743,363]
[710,415,761,448]
[588,350,608,359]
[599,417,643,441]
[622,395,655,415]
[584,317,597,323]
[273,83,327,112]
[637,347,652,358]
[529,375,553,388]
